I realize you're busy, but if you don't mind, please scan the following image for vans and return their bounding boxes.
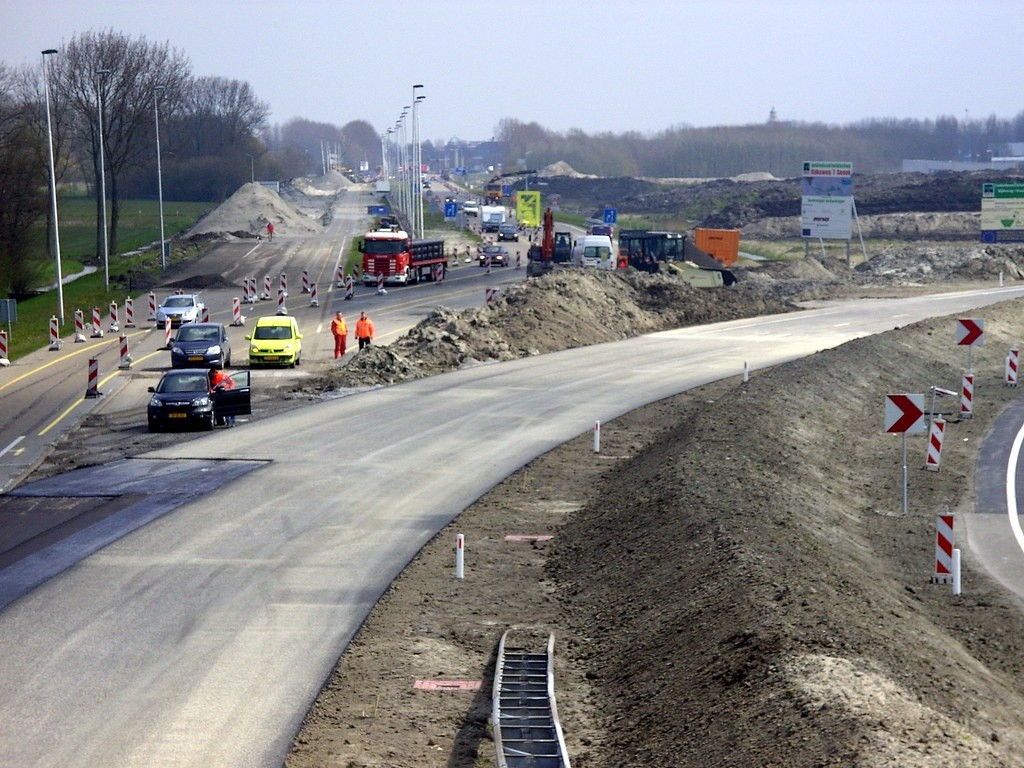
[586,225,614,242]
[580,235,614,272]
[245,315,304,369]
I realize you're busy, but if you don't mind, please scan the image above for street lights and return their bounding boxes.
[153,84,165,270]
[382,84,425,239]
[41,49,66,329]
[96,69,112,291]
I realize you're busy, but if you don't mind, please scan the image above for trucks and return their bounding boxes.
[478,206,506,231]
[483,183,503,204]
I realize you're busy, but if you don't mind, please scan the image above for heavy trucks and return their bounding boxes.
[358,225,449,285]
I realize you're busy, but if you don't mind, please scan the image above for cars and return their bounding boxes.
[146,367,252,429]
[479,244,509,266]
[445,196,455,202]
[497,225,519,242]
[169,324,231,370]
[422,182,431,188]
[156,294,205,327]
[458,200,477,217]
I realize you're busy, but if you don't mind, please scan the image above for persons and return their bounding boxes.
[330,310,348,359]
[267,222,274,242]
[208,368,238,428]
[355,311,374,351]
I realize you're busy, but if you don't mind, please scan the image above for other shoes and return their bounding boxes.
[226,423,235,428]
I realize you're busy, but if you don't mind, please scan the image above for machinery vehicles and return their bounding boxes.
[526,208,572,277]
[618,229,689,273]
